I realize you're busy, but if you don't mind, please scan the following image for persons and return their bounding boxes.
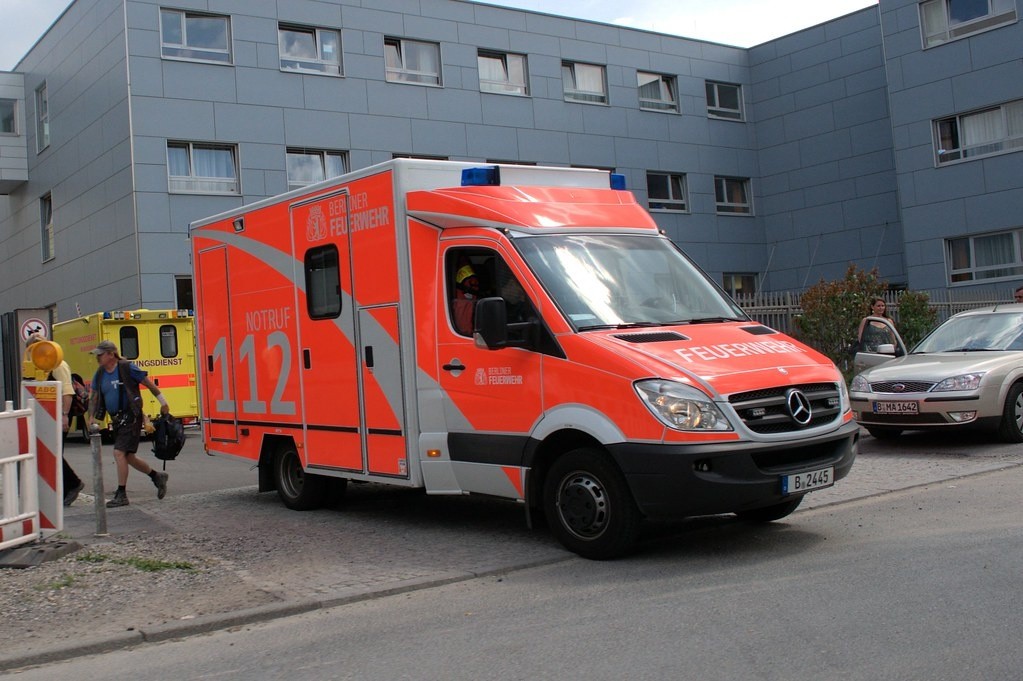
[451,265,480,338]
[1014,286,1023,303]
[88,341,169,507]
[21,332,85,506]
[858,296,897,355]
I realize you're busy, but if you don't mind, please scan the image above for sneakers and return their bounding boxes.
[63,481,85,506]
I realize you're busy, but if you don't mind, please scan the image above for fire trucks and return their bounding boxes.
[187,158,861,561]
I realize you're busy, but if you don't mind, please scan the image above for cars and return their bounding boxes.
[849,301,1023,443]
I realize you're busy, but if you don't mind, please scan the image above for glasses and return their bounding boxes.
[1014,295,1023,299]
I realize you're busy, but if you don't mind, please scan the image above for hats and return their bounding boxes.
[89,341,117,355]
[456,265,476,283]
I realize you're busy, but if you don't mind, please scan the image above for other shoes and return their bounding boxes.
[152,472,169,499]
[106,490,129,508]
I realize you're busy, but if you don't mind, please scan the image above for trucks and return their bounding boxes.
[51,309,200,445]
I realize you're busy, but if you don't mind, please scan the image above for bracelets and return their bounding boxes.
[62,412,68,416]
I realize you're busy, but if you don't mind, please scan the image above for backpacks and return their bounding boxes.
[71,373,89,440]
[145,414,186,471]
